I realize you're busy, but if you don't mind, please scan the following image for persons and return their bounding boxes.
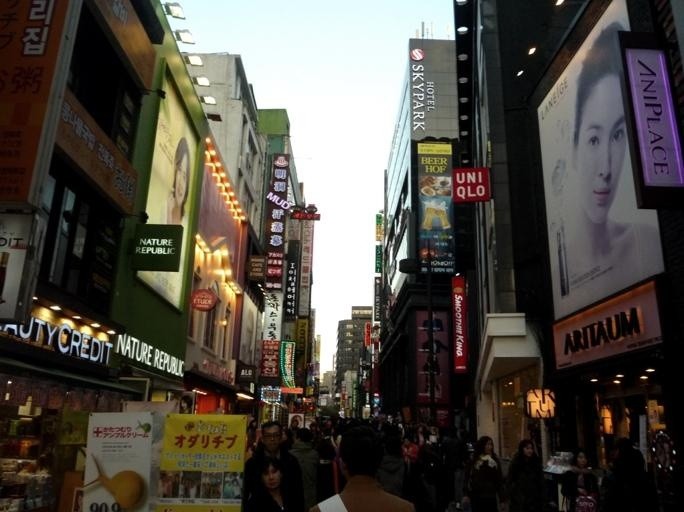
[240,410,657,511]
[160,136,192,228]
[551,21,664,320]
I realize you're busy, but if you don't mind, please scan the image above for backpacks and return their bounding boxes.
[574,470,599,512]
